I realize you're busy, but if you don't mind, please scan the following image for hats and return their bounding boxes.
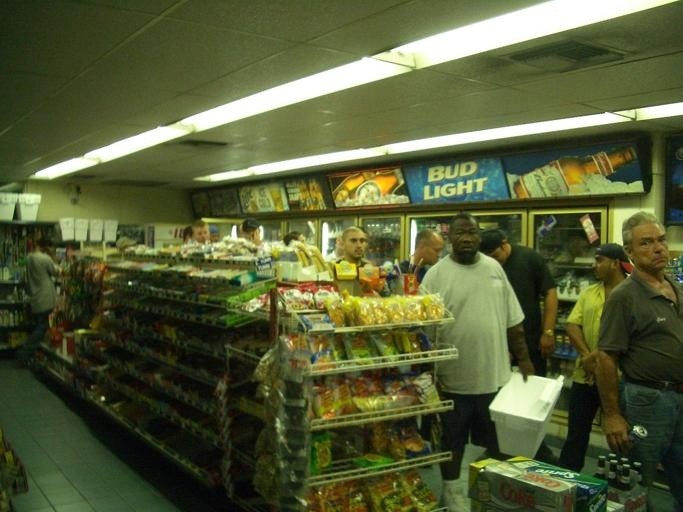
[596,242,634,274]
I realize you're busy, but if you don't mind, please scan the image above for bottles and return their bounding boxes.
[330,169,404,208]
[297,177,313,211]
[592,453,646,493]
[308,177,326,210]
[553,332,580,377]
[512,145,638,199]
[362,220,400,265]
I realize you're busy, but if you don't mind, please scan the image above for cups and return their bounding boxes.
[268,183,284,213]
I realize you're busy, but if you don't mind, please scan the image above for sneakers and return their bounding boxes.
[443,479,470,511]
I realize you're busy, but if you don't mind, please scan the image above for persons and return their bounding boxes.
[479,230,557,377]
[560,244,635,472]
[326,226,369,268]
[595,212,683,512]
[183,221,219,244]
[237,219,262,246]
[398,230,444,283]
[420,216,534,512]
[285,231,304,246]
[14,237,64,367]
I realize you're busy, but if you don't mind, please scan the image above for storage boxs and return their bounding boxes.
[18,192,44,223]
[488,370,566,460]
[0,192,18,223]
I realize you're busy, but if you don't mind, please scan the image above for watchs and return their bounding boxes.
[543,329,554,337]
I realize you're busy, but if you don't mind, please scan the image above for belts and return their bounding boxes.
[627,377,682,392]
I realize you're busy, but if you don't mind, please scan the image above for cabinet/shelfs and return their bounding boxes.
[1,221,459,512]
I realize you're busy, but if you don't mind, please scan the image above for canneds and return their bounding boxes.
[628,424,650,448]
